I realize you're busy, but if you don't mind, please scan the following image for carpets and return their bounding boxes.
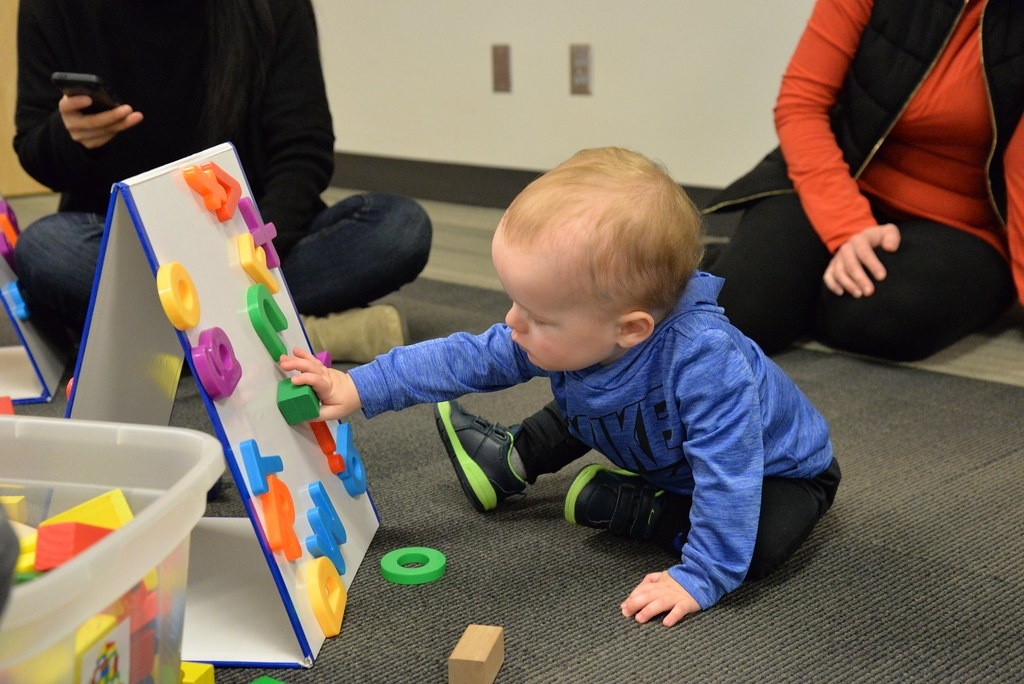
[1,278,1024,683]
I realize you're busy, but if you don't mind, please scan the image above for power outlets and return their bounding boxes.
[491,44,512,91]
[568,43,591,94]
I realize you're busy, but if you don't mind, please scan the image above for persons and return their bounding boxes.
[280,144,842,636]
[706,0,1024,368]
[9,0,434,396]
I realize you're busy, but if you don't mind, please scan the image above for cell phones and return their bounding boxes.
[51,72,113,115]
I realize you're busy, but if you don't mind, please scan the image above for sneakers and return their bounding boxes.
[562,464,669,541]
[433,396,525,511]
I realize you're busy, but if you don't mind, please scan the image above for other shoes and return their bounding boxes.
[298,299,408,364]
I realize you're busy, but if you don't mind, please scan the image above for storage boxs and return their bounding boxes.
[0,412,227,684]
